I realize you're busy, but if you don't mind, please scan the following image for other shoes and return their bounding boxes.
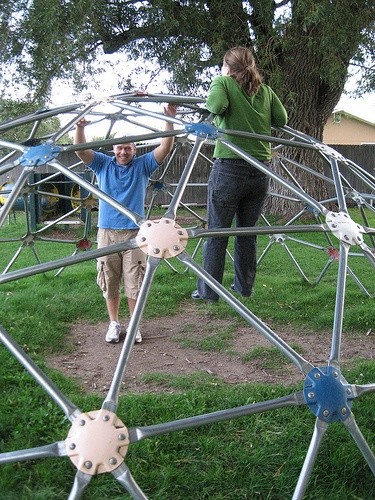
[231,281,236,291]
[191,290,201,300]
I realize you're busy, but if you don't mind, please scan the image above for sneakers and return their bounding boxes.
[106,320,121,343]
[126,326,142,343]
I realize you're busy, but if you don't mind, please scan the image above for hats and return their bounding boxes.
[113,131,136,146]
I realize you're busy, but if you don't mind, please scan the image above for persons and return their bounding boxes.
[72,101,179,344]
[190,47,288,301]
